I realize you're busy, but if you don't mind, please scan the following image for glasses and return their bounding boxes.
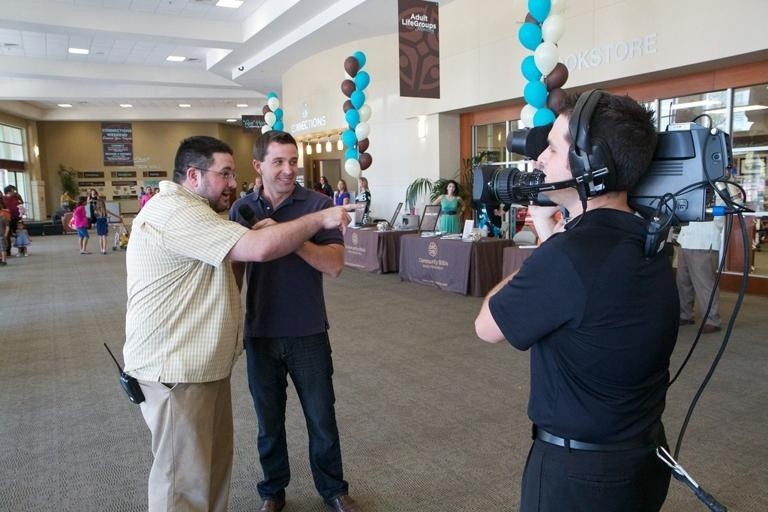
[337,131,343,150]
[315,135,321,153]
[325,135,332,152]
[306,137,313,154]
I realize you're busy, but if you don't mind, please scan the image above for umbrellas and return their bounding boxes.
[701,324,719,333]
[260,499,285,512]
[325,493,361,512]
[680,318,694,325]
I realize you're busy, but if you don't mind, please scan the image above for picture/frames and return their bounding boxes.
[399,233,515,297]
[502,246,536,280]
[344,225,418,274]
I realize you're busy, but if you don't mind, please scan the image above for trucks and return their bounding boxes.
[261,89,284,134]
[341,51,373,179]
[518,0,571,129]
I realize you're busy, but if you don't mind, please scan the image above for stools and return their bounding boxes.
[535,427,658,451]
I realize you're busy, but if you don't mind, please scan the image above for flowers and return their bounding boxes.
[512,174,596,200]
[237,204,260,226]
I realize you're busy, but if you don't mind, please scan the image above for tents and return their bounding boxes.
[569,89,616,197]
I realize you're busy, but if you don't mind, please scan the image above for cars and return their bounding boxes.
[410,208,416,215]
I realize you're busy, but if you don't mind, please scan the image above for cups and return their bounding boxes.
[187,165,237,181]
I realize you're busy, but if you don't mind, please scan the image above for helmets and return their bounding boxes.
[361,224,377,227]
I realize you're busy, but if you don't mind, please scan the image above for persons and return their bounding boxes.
[0,184,33,264]
[673,178,733,333]
[332,179,350,206]
[354,177,373,226]
[430,179,466,234]
[227,131,368,512]
[472,88,682,512]
[238,175,265,200]
[316,176,334,201]
[58,189,130,254]
[133,186,160,216]
[123,135,366,511]
[468,200,511,237]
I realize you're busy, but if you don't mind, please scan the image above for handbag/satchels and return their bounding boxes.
[460,209,464,214]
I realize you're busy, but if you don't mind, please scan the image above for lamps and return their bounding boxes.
[61,213,78,235]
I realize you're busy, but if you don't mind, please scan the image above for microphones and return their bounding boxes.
[25,220,63,235]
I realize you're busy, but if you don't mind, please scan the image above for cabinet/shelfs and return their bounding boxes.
[363,214,368,225]
